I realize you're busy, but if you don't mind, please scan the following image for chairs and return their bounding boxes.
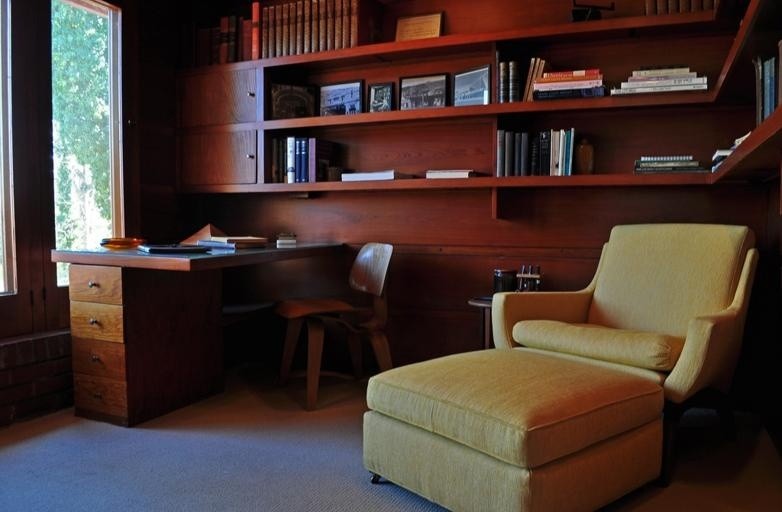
[271,241,395,414]
[491,219,761,490]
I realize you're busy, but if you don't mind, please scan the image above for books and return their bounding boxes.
[427,169,477,179]
[496,127,576,179]
[211,1,374,64]
[335,169,411,181]
[267,136,342,184]
[631,154,711,175]
[135,236,270,256]
[751,55,777,127]
[711,147,733,173]
[497,57,709,104]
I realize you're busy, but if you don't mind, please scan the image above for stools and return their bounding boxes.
[359,347,665,512]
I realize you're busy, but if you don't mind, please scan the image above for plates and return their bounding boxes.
[101,238,146,250]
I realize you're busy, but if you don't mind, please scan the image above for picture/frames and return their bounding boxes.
[397,72,452,110]
[267,79,317,119]
[367,82,395,113]
[394,12,444,43]
[317,78,366,117]
[452,62,492,106]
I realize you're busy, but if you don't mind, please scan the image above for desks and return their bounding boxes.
[467,298,492,350]
[48,241,350,428]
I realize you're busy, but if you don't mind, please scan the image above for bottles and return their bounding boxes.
[492,269,516,295]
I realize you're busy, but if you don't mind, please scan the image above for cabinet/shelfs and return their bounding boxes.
[495,10,712,187]
[712,0,782,187]
[264,29,494,193]
[172,60,292,194]
